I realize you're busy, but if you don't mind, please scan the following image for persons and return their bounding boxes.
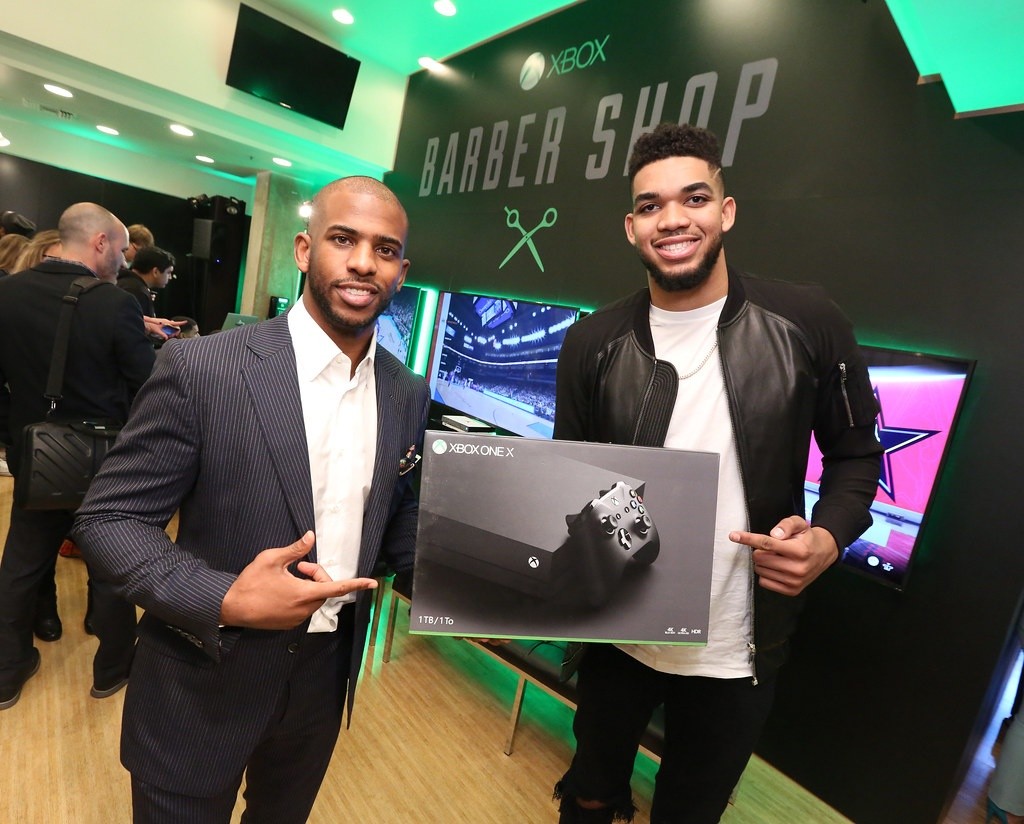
[69,176,510,824]
[0,203,201,711]
[552,124,885,824]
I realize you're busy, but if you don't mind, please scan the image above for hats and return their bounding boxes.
[2,211,37,238]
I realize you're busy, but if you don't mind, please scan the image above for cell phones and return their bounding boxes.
[147,326,180,342]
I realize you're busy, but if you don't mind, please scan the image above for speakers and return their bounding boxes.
[196,195,245,335]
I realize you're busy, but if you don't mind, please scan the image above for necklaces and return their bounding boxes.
[679,340,717,380]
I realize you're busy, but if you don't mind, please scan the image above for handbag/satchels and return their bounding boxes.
[15,416,124,510]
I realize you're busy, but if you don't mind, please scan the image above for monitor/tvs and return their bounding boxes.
[372,284,422,368]
[424,290,580,439]
[224,2,363,130]
[801,345,979,598]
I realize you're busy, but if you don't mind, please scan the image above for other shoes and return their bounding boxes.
[58,537,80,558]
[84,608,92,634]
[0,646,40,710]
[34,597,62,642]
[91,677,128,698]
